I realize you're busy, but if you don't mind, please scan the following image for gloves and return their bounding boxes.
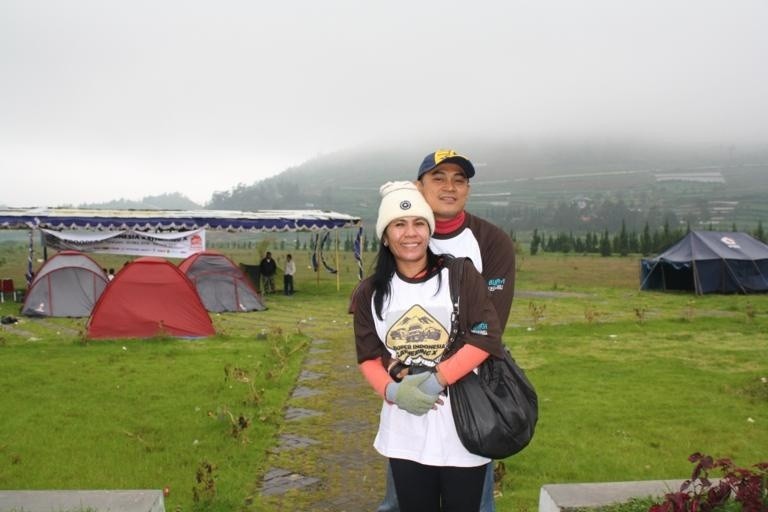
[385,371,444,414]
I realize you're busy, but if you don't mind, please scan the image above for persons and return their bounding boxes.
[108,269,114,281]
[284,254,296,295]
[260,252,276,294]
[353,180,503,512]
[377,152,515,512]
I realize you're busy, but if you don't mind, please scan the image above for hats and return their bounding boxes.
[377,181,435,239]
[418,150,474,177]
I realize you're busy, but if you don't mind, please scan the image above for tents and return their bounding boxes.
[640,226,768,295]
[177,253,268,312]
[20,250,110,317]
[86,256,215,338]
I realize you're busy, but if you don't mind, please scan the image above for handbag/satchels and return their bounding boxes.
[442,347,538,458]
